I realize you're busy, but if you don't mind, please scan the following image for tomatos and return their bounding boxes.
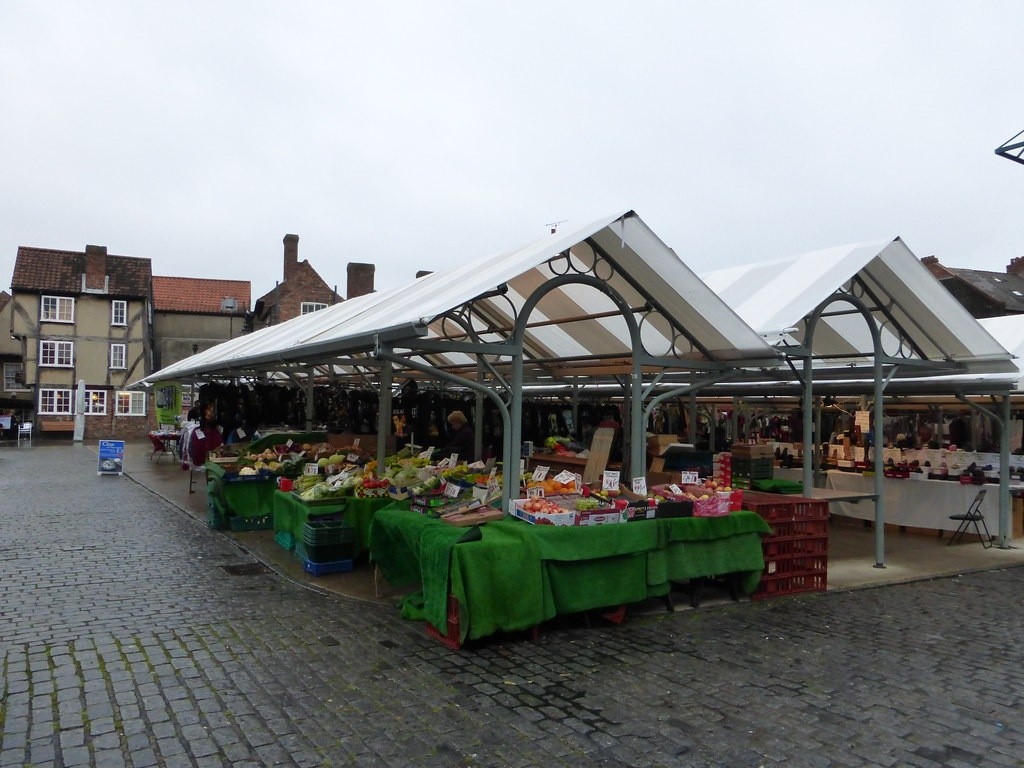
[362,478,388,489]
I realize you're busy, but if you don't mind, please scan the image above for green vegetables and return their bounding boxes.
[371,456,467,495]
[236,456,317,480]
[292,474,332,500]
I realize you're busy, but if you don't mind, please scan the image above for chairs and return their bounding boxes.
[946,489,993,549]
[18,422,33,446]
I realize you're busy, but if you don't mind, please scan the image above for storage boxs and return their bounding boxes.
[508,434,773,525]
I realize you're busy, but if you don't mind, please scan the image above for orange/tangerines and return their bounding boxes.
[527,479,575,494]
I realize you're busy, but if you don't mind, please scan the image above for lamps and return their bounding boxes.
[821,398,838,408]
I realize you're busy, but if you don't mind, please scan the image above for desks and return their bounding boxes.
[206,453,768,641]
[773,465,1014,541]
[147,431,182,465]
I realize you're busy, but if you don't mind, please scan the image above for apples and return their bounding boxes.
[522,495,569,515]
[591,480,708,501]
[703,479,731,493]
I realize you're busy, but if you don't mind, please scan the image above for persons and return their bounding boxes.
[433,410,474,464]
[599,411,620,430]
[187,400,200,421]
[10,415,17,432]
[715,419,725,450]
[422,421,447,448]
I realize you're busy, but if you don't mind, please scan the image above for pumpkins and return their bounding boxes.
[249,448,277,461]
